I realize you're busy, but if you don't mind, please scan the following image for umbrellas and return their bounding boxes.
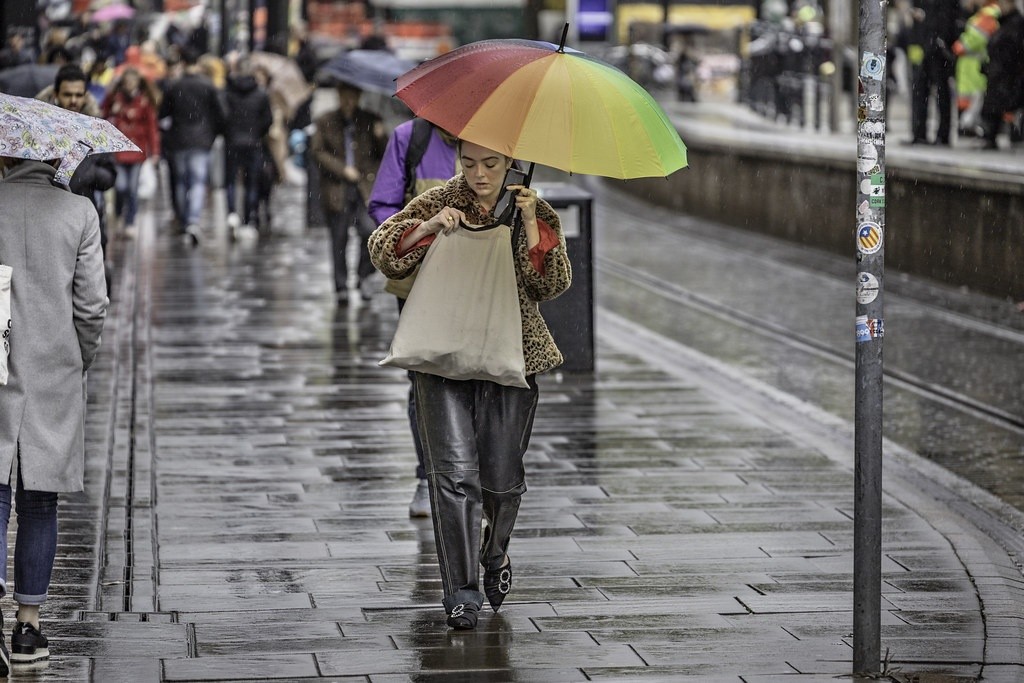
[391,21,691,259]
[316,47,415,104]
[0,92,143,186]
[663,24,710,44]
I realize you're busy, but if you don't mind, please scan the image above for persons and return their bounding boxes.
[33,64,101,117]
[367,118,572,630]
[622,1,1024,150]
[0,156,110,677]
[0,1,312,246]
[307,82,386,306]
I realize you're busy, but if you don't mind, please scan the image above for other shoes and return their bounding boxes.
[187,224,201,247]
[124,227,136,242]
[115,219,126,238]
[337,290,350,307]
[410,480,434,516]
[358,281,372,300]
[229,214,238,242]
[447,603,477,629]
[482,554,512,614]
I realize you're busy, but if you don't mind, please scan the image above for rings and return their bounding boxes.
[449,217,452,221]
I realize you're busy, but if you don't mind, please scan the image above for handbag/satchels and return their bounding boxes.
[259,144,282,186]
[378,190,531,392]
[139,160,158,200]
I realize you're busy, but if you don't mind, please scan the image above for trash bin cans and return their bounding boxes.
[530,182,597,373]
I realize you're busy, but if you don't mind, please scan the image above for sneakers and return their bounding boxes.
[10,621,50,663]
[0,629,9,677]
